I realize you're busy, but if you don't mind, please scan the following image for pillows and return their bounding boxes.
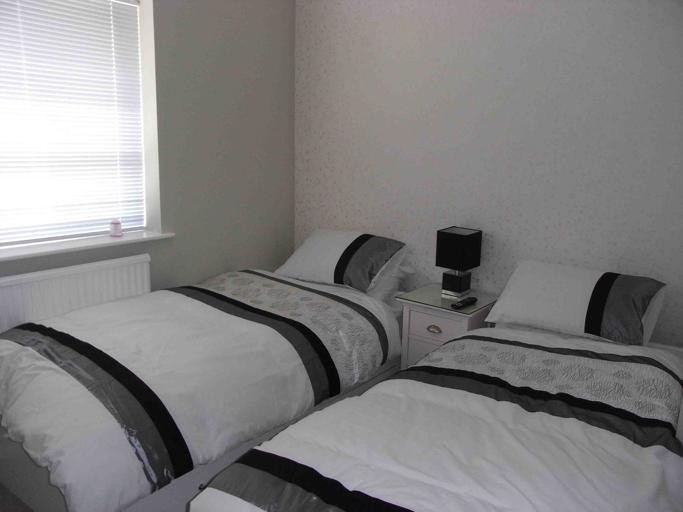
[274,229,408,293]
[484,259,668,347]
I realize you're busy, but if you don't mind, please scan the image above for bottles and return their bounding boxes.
[109,218,124,237]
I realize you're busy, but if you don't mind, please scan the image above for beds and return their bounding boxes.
[0,270,402,512]
[187,326,682,511]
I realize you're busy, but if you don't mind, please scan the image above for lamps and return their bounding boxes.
[435,226,482,303]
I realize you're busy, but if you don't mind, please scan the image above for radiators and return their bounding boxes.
[0,253,151,334]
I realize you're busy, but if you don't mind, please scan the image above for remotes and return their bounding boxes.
[451,297,478,309]
